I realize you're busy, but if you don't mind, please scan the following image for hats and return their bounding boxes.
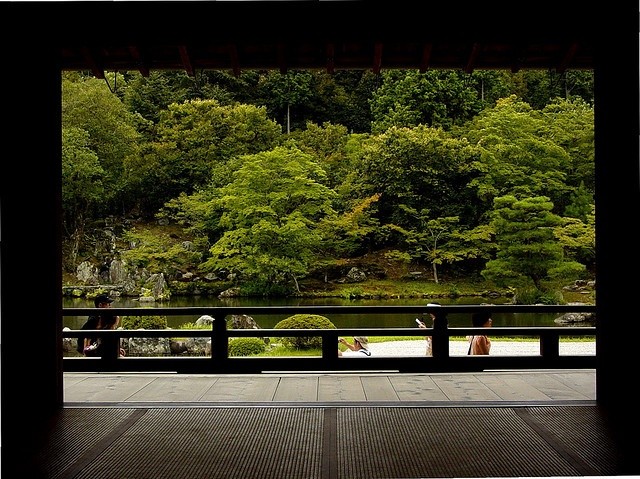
[354,336,369,351]
[94,293,115,304]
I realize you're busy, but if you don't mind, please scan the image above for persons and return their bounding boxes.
[338,336,371,356]
[467,314,492,355]
[76,293,126,357]
[418,302,448,356]
[83,314,119,357]
